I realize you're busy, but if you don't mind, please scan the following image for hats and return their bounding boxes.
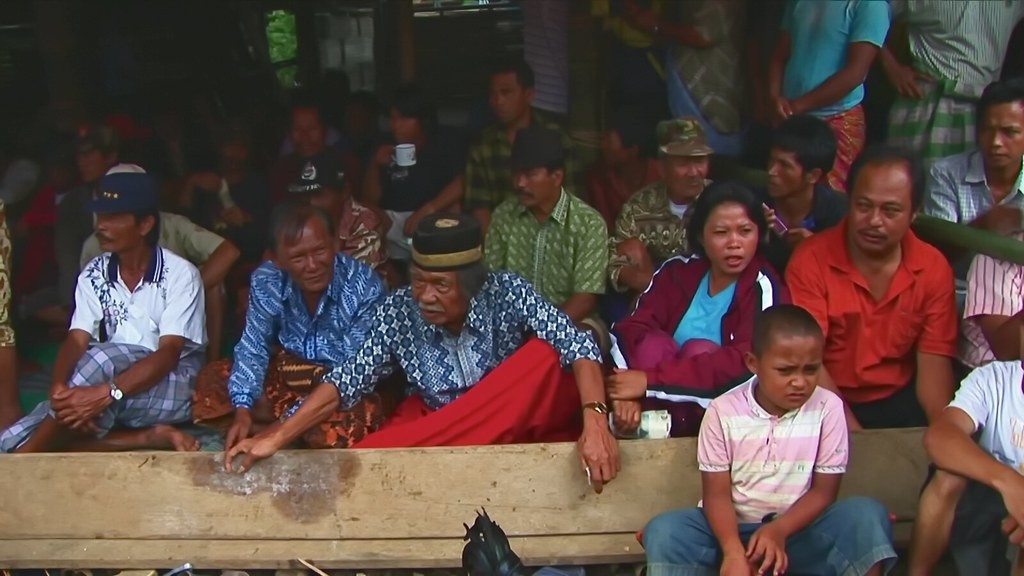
[654,116,714,157]
[107,162,147,176]
[410,212,485,272]
[89,172,154,212]
[506,127,570,170]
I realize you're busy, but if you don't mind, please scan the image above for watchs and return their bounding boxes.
[582,401,608,415]
[106,379,125,403]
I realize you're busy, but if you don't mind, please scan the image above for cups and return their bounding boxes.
[390,143,417,166]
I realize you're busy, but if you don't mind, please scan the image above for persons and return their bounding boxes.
[0,0,1024,496]
[643,304,898,576]
[905,361,1024,576]
